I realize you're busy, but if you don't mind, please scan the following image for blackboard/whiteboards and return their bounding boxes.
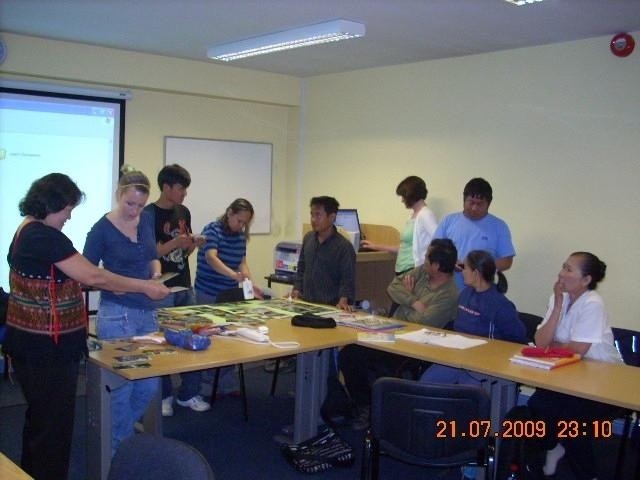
[162,135,273,236]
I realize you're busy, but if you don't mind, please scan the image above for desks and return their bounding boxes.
[82,294,640,479]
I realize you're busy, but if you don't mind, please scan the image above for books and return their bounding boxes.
[391,325,488,351]
[514,352,581,366]
[509,357,581,371]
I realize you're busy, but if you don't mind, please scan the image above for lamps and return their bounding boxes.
[207,19,366,63]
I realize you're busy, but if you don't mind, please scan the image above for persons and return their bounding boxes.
[7,174,173,479]
[429,177,516,297]
[334,240,457,432]
[529,251,628,480]
[282,194,357,401]
[359,176,438,319]
[139,164,212,419]
[410,250,530,403]
[192,197,270,402]
[81,164,164,463]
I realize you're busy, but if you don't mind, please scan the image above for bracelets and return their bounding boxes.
[151,272,162,279]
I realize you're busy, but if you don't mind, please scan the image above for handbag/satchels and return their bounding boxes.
[284,424,355,474]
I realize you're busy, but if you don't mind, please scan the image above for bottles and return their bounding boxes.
[502,463,520,480]
[243,277,254,300]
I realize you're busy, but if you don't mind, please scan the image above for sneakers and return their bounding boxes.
[352,405,371,431]
[176,394,211,412]
[215,394,224,401]
[161,396,174,416]
[231,392,241,396]
[280,424,295,436]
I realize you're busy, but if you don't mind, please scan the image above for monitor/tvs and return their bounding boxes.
[333,209,360,234]
